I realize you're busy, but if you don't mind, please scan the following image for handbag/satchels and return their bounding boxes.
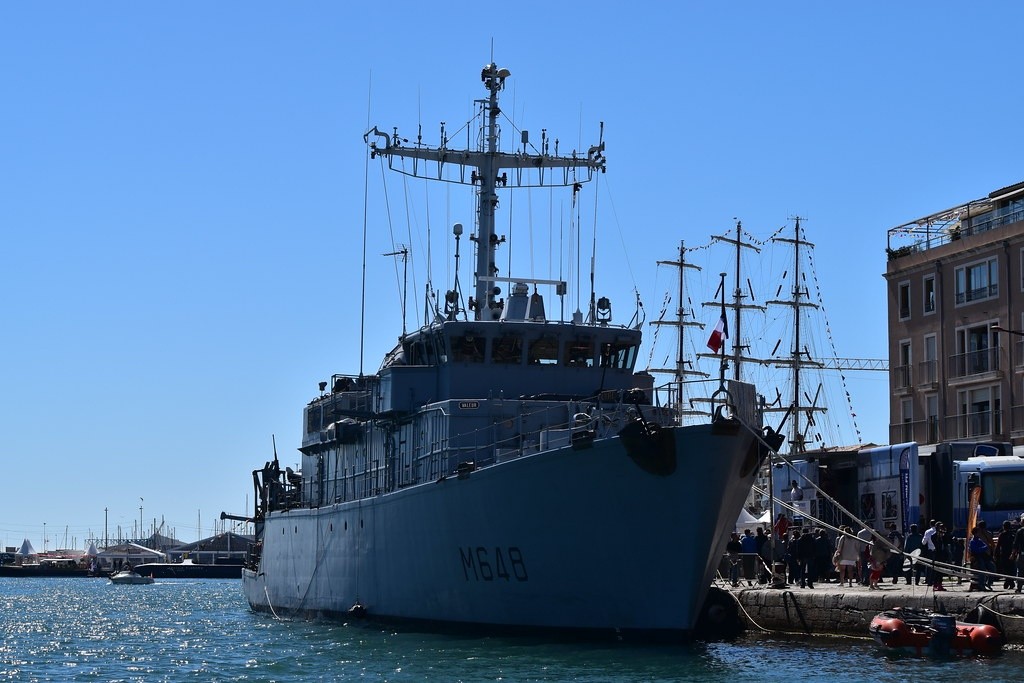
[832,551,841,566]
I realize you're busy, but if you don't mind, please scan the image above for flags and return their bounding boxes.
[707,314,728,353]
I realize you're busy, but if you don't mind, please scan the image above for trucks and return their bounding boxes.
[770,436,1024,552]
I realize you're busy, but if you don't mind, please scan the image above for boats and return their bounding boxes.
[109,570,155,585]
[867,604,1005,656]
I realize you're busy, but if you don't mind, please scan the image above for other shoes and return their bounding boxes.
[801,584,806,588]
[731,583,739,587]
[915,582,918,585]
[1015,589,1021,593]
[978,589,989,592]
[933,584,946,591]
[1009,584,1015,589]
[807,583,814,589]
[905,582,911,585]
[870,585,883,590]
[1003,583,1008,589]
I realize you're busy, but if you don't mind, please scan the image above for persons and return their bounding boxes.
[726,514,1024,593]
[837,527,856,587]
[791,480,803,501]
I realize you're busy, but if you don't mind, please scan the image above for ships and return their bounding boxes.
[220,33,785,636]
